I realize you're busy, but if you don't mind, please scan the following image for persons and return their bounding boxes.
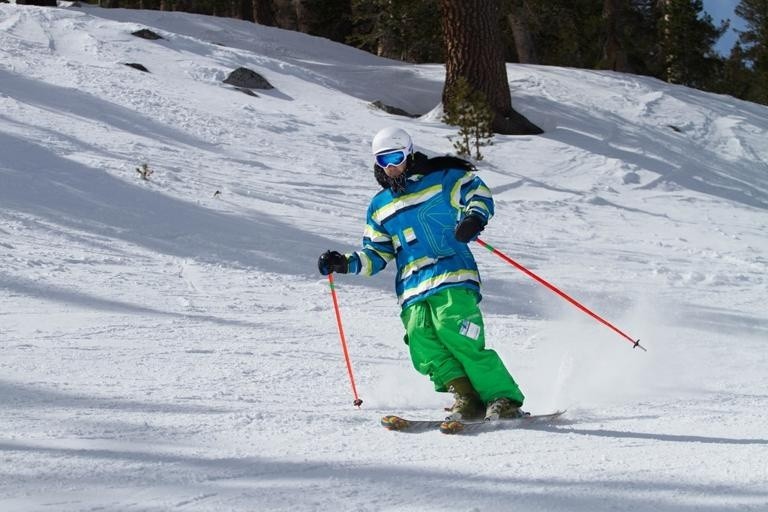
[317,126,525,423]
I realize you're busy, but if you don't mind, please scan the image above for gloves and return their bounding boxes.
[318,250,348,275]
[454,213,488,243]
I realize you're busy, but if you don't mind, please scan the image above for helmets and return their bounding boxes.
[372,127,413,158]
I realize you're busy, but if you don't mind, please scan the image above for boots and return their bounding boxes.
[444,376,486,418]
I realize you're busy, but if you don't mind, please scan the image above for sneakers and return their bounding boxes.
[485,399,523,418]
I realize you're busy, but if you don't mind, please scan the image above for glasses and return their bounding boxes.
[374,150,407,169]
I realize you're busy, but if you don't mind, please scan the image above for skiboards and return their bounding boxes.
[381,410,567,433]
